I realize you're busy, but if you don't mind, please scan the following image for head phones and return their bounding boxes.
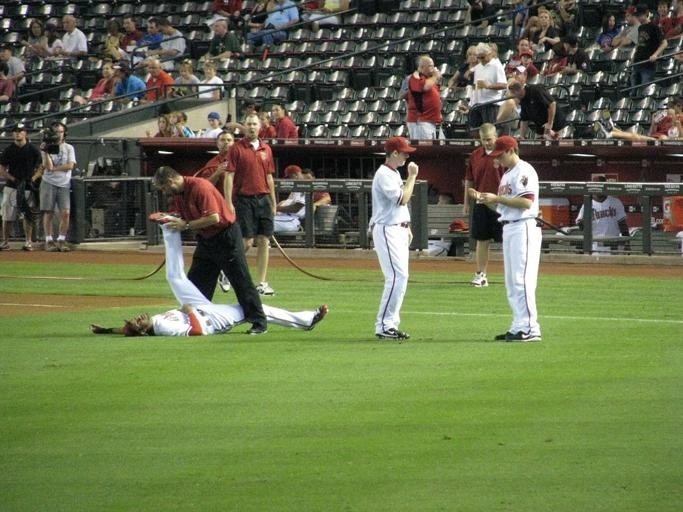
[51,122,69,137]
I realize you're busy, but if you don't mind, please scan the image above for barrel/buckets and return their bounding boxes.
[663,196,683,232]
[317,205,338,229]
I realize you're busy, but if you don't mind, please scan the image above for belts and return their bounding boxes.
[401,223,408,227]
[501,219,520,226]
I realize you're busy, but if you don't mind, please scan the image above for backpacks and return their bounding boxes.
[273,211,301,236]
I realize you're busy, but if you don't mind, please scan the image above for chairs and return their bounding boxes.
[1,0,683,255]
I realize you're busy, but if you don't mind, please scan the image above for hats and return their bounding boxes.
[489,136,518,157]
[384,137,417,153]
[208,112,220,119]
[10,123,24,131]
[283,165,302,178]
[450,218,469,230]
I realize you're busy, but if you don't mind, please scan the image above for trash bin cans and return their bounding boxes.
[90,204,113,237]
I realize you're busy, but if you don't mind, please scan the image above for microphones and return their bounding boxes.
[63,137,66,140]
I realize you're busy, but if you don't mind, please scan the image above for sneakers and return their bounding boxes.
[247,326,267,335]
[218,270,230,292]
[375,327,410,341]
[44,237,71,252]
[593,108,615,139]
[471,271,489,287]
[495,328,542,341]
[254,282,274,296]
[309,304,328,330]
[148,212,181,223]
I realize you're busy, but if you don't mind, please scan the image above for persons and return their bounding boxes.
[468,135,543,341]
[90,212,330,336]
[152,165,269,336]
[368,136,419,339]
[463,123,509,287]
[218,112,279,297]
